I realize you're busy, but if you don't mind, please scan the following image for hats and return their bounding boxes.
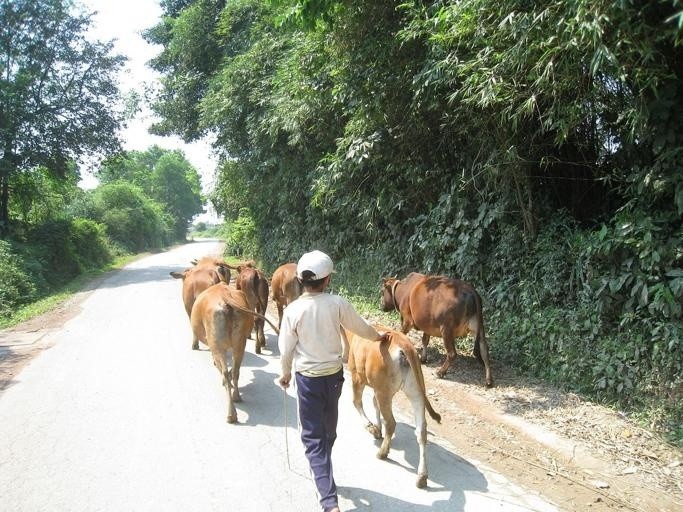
[296,250,337,282]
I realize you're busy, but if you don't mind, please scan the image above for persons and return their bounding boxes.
[277,250,390,512]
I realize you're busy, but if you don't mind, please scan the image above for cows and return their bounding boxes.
[342,311,441,489]
[170,267,225,350]
[191,281,279,424]
[380,270,493,389]
[191,257,236,286]
[236,263,269,354]
[271,262,304,329]
